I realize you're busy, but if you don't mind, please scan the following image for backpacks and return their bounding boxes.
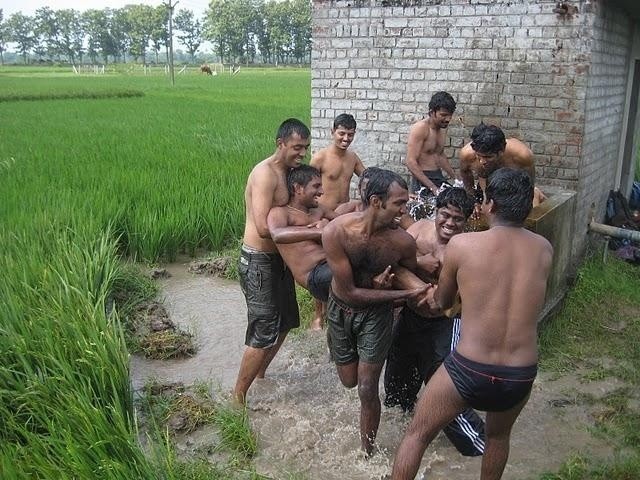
[630,182,640,209]
[607,189,632,227]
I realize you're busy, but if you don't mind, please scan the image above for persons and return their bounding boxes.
[380,167,553,480]
[264,162,461,321]
[457,119,547,219]
[324,169,420,460]
[232,116,311,408]
[382,184,486,458]
[307,166,382,230]
[402,90,458,214]
[309,112,367,332]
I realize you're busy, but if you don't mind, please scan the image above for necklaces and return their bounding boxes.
[287,203,312,217]
